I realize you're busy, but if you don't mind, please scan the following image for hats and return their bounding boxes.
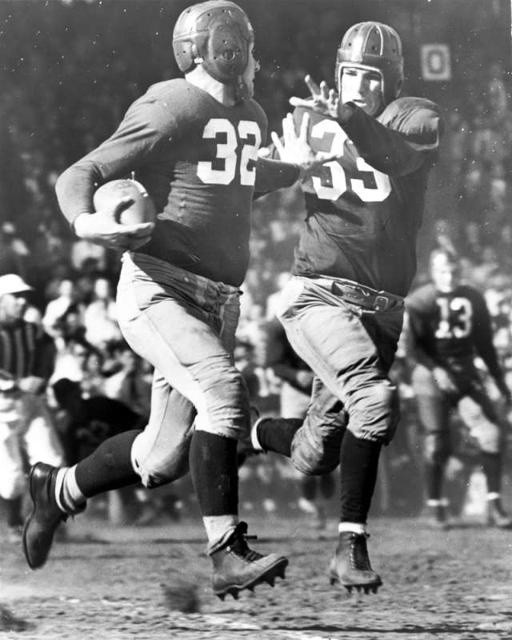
[0,273,32,294]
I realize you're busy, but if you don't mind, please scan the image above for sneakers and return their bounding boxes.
[488,501,512,527]
[238,405,261,465]
[23,461,59,570]
[329,532,384,594]
[210,537,289,600]
[424,506,448,530]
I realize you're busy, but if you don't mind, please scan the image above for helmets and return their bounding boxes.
[173,0,255,84]
[336,22,404,105]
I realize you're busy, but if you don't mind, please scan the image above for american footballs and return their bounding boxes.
[92,178,156,225]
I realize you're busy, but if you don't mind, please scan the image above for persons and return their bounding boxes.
[21,1,289,600]
[0,0,512,542]
[245,21,444,598]
